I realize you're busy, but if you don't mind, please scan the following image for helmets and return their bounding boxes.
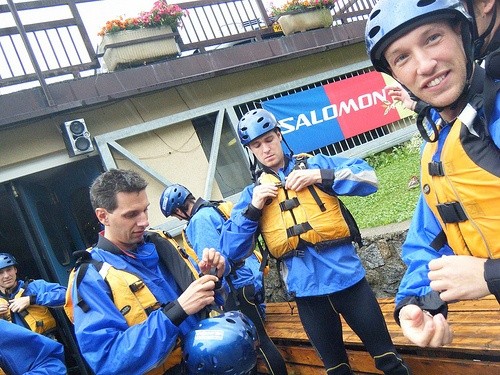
[238,109,277,146]
[183,311,257,375]
[0,253,17,270]
[365,0,473,72]
[160,184,192,217]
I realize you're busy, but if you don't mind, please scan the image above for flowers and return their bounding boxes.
[97,0,189,36]
[269,0,337,32]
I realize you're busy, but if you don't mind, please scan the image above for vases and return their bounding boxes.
[100,24,180,73]
[278,9,334,36]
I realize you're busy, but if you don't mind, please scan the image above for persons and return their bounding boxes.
[364,0,500,349]
[160,184,288,375]
[458,0,500,60]
[0,318,66,375]
[63,167,230,375]
[0,253,67,340]
[216,109,410,374]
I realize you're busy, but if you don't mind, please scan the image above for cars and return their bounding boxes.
[163,0,297,59]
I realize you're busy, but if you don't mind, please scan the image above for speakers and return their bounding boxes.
[59,117,94,158]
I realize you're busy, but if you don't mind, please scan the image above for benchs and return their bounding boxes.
[257,294,500,375]
